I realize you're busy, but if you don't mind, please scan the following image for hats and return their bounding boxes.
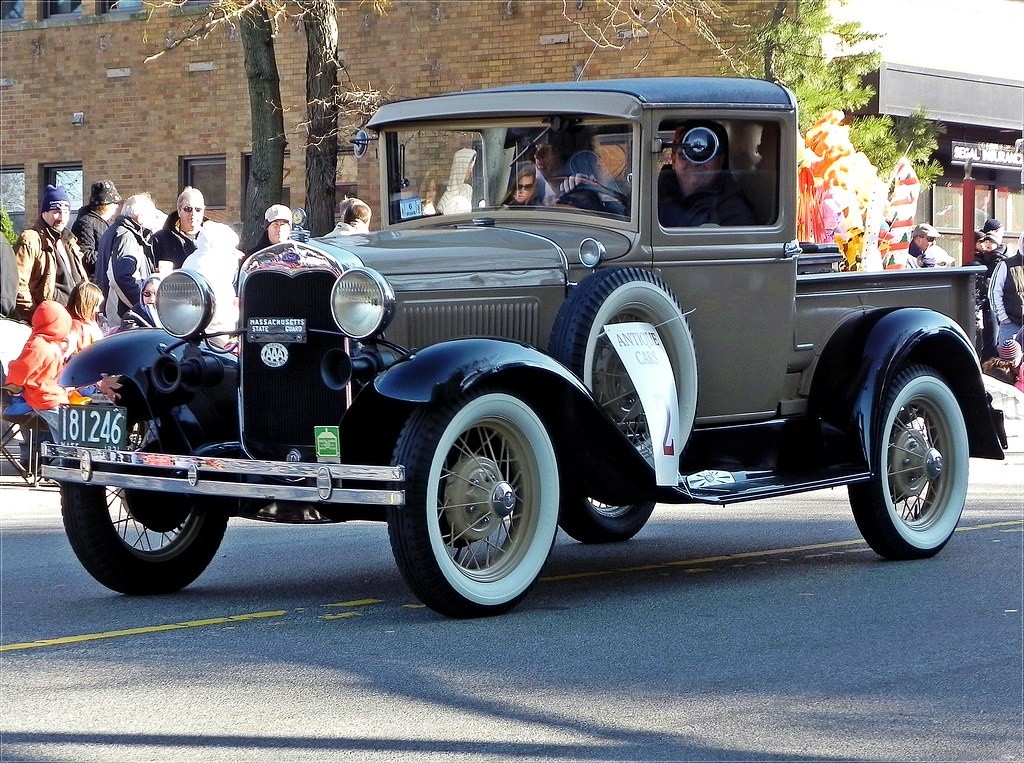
[88,179,123,206]
[912,223,942,239]
[999,339,1023,362]
[262,204,293,231]
[41,183,71,212]
[974,219,1005,247]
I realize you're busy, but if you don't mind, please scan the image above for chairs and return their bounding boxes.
[0,362,98,488]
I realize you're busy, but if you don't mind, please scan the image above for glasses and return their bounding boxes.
[139,290,157,298]
[514,184,533,191]
[180,205,203,213]
[916,235,936,242]
[673,149,688,162]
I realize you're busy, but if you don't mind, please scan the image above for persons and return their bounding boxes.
[526,124,595,204]
[419,169,446,214]
[558,120,757,227]
[500,165,537,207]
[324,197,372,237]
[254,204,294,251]
[438,148,477,214]
[908,223,955,268]
[0,179,244,464]
[974,219,1024,385]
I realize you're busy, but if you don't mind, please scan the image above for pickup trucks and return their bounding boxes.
[39,77,1008,618]
